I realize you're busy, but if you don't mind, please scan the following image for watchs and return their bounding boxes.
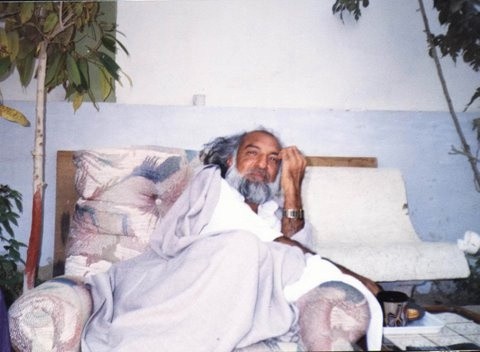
[283,209,304,220]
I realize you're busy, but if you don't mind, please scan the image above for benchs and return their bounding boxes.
[273,166,471,297]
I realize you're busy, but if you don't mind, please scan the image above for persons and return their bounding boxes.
[80,129,382,352]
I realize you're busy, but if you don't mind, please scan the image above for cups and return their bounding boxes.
[376,291,424,327]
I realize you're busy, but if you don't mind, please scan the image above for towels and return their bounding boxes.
[81,165,383,351]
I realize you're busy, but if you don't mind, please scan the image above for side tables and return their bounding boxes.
[381,306,480,352]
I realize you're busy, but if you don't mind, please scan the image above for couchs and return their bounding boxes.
[8,146,371,352]
